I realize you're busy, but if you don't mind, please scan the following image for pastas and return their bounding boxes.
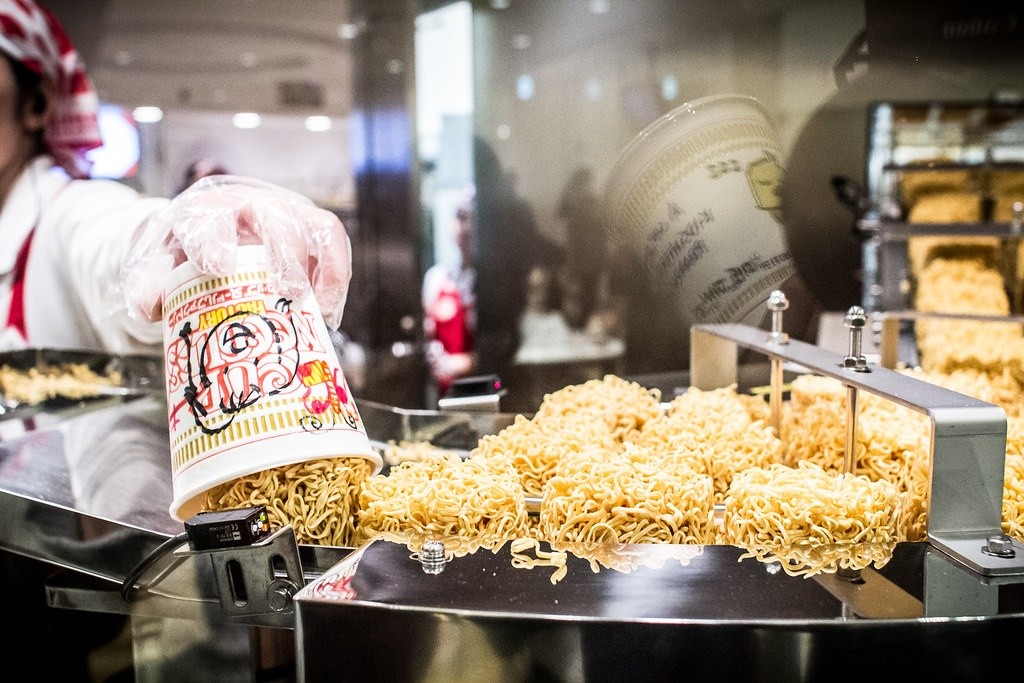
[197,197,1024,581]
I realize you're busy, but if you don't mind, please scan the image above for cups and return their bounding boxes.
[158,243,383,528]
[593,97,811,357]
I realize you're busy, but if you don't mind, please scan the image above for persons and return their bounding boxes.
[170,157,209,204]
[0,0,354,357]
[466,125,670,398]
